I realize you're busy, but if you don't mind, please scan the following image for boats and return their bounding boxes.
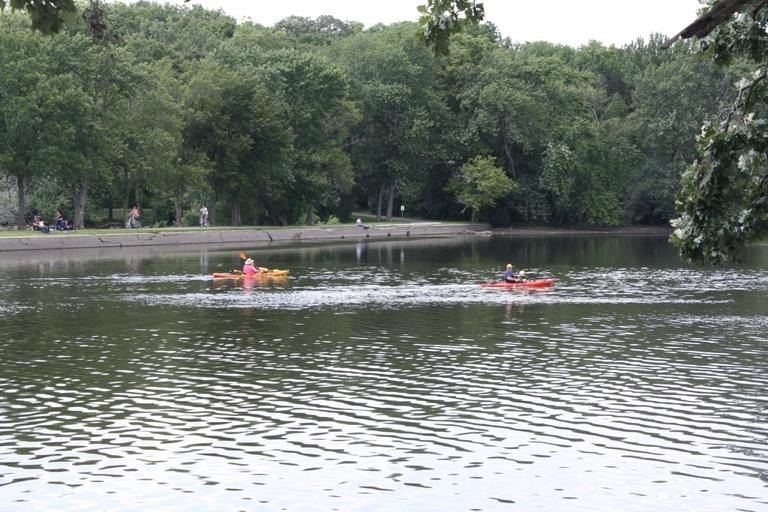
[213,269,290,279]
[481,278,553,288]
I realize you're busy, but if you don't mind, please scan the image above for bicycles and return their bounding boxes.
[126,214,141,229]
[200,215,209,227]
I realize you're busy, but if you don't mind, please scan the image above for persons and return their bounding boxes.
[128,205,139,224]
[242,257,259,273]
[31,209,73,234]
[503,263,521,282]
[200,203,208,226]
[357,216,371,229]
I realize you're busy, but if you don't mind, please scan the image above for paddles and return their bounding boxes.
[240,252,259,270]
[513,269,524,288]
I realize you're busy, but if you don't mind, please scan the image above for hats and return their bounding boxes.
[244,258,255,265]
